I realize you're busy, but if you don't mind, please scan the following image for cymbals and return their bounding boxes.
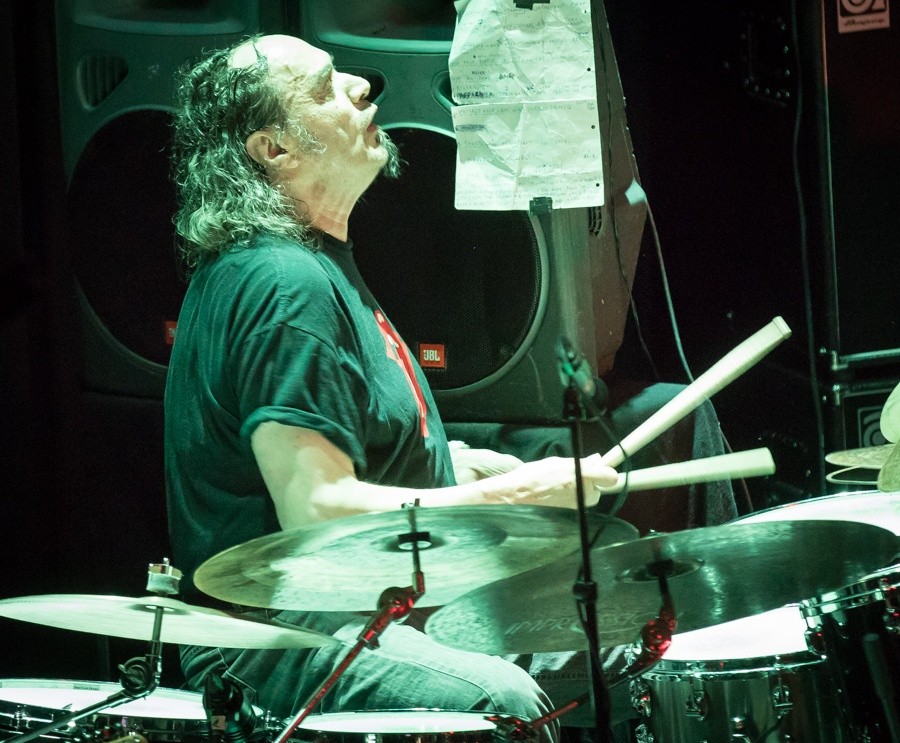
[191,506,642,614]
[0,593,342,649]
[877,440,900,492]
[825,444,897,469]
[424,519,900,652]
[880,381,900,443]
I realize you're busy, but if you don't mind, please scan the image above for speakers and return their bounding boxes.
[53,0,900,518]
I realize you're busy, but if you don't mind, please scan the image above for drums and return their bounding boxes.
[623,490,900,743]
[281,708,532,743]
[797,563,900,743]
[0,677,264,743]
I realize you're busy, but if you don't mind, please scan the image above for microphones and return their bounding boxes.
[199,674,257,743]
[554,338,610,418]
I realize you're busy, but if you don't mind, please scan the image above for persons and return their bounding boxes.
[165,31,616,743]
